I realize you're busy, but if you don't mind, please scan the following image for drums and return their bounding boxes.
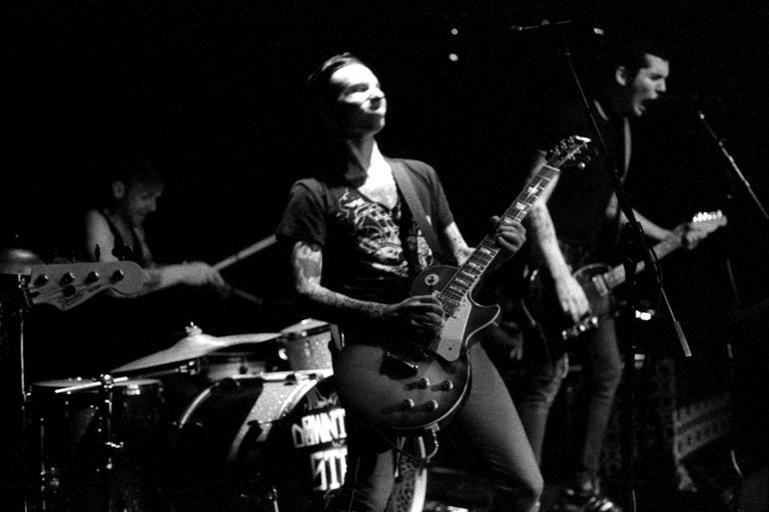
[282,319,333,379]
[199,351,267,382]
[164,371,427,511]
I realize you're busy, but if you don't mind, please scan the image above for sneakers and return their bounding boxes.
[555,477,628,511]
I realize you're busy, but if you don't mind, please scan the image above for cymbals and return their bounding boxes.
[111,333,280,373]
[36,378,160,389]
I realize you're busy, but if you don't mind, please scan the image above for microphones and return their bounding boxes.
[661,94,722,109]
[508,20,572,40]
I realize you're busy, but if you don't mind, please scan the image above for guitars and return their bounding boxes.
[334,135,597,437]
[523,212,726,346]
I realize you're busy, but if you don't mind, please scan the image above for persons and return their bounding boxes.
[521,37,709,512]
[262,50,547,512]
[59,152,226,381]
